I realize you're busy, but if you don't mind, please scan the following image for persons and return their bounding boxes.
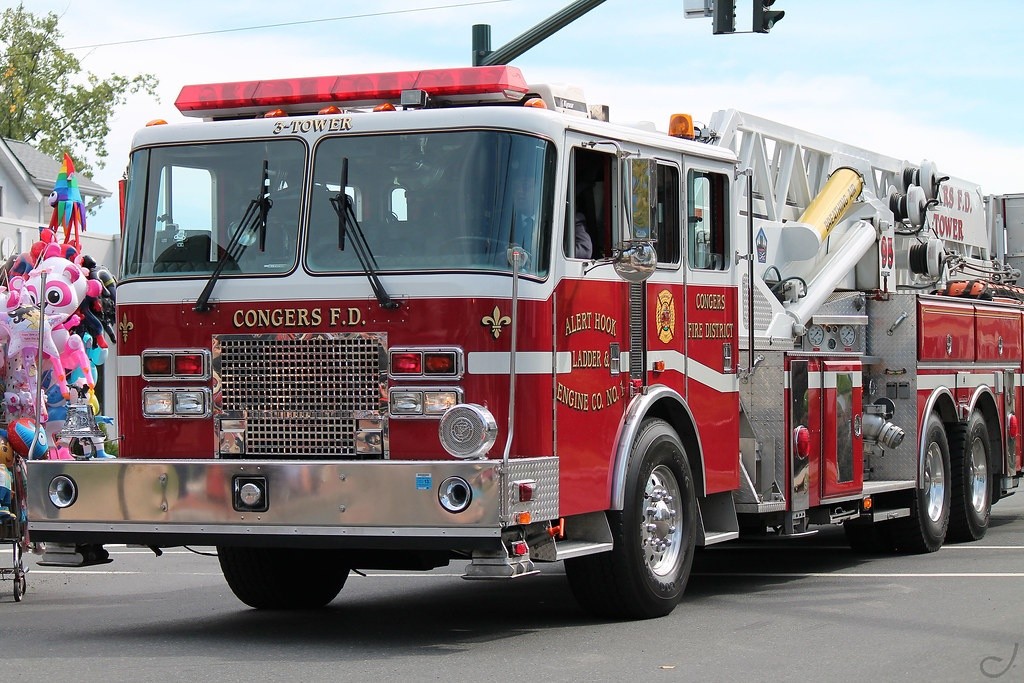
[483,156,594,269]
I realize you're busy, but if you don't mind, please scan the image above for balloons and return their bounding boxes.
[0,428,17,519]
[0,229,117,458]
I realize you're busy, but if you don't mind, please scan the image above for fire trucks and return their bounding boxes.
[23,55,1024,622]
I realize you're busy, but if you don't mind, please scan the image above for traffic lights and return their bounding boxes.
[752,1,785,33]
